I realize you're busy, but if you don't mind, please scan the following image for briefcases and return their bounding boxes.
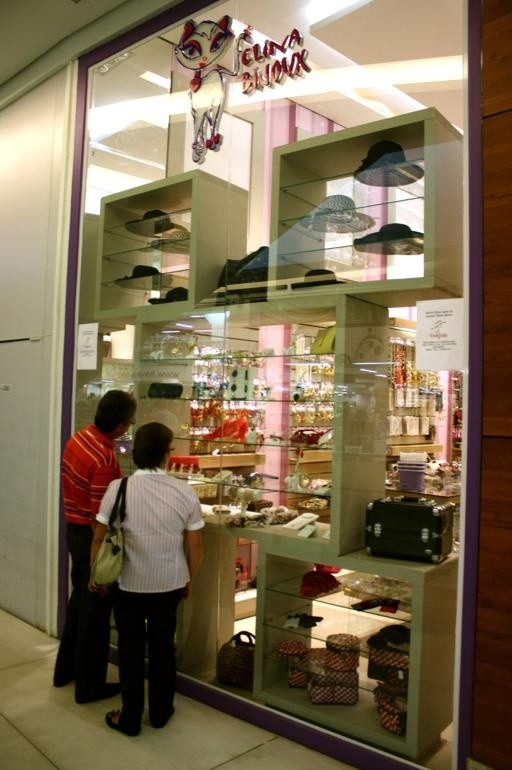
[366,494,455,564]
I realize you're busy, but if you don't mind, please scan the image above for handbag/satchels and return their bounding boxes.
[224,510,269,529]
[278,634,410,736]
[149,383,183,398]
[216,630,257,692]
[215,246,269,305]
[259,506,299,524]
[310,325,337,354]
[88,477,127,590]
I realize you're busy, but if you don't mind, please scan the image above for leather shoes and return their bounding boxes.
[76,683,121,704]
[52,673,82,687]
[152,707,175,729]
[106,711,135,737]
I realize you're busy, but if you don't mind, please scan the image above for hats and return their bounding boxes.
[151,230,190,254]
[353,140,424,188]
[300,195,375,234]
[126,209,175,238]
[114,266,173,290]
[285,269,347,290]
[355,224,425,255]
[148,287,188,304]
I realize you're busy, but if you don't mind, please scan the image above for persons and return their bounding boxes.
[53,388,137,706]
[86,421,208,738]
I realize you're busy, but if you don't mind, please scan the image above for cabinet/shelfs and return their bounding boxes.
[78,98,466,761]
